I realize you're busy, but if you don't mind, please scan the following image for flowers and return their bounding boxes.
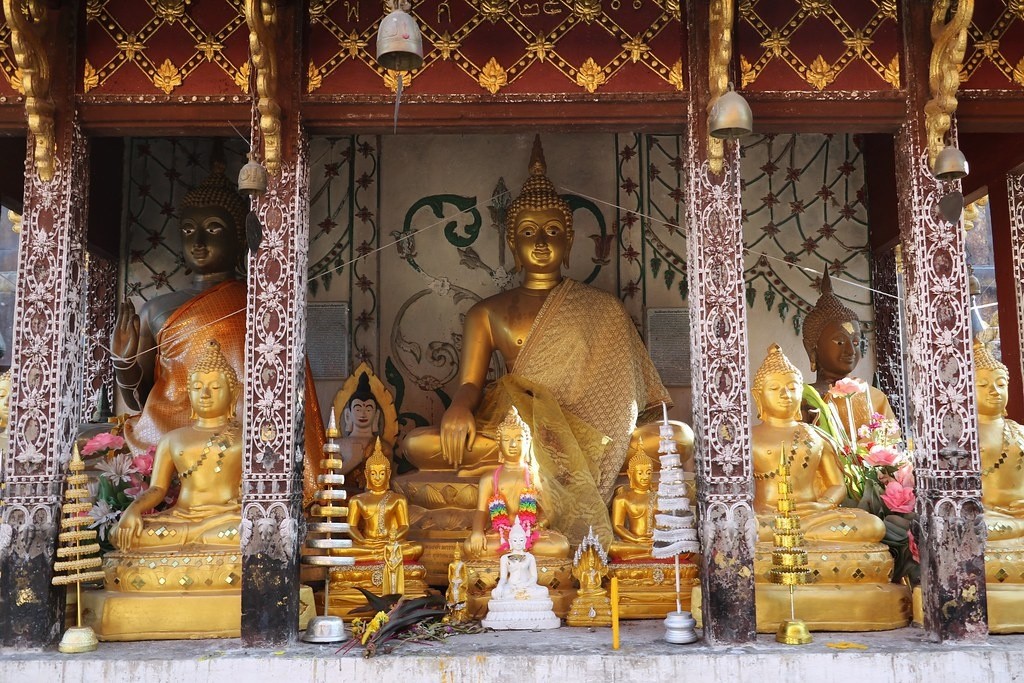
[72,432,182,585]
[802,377,921,584]
[334,587,495,661]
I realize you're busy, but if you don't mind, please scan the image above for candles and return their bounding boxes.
[611,569,620,649]
